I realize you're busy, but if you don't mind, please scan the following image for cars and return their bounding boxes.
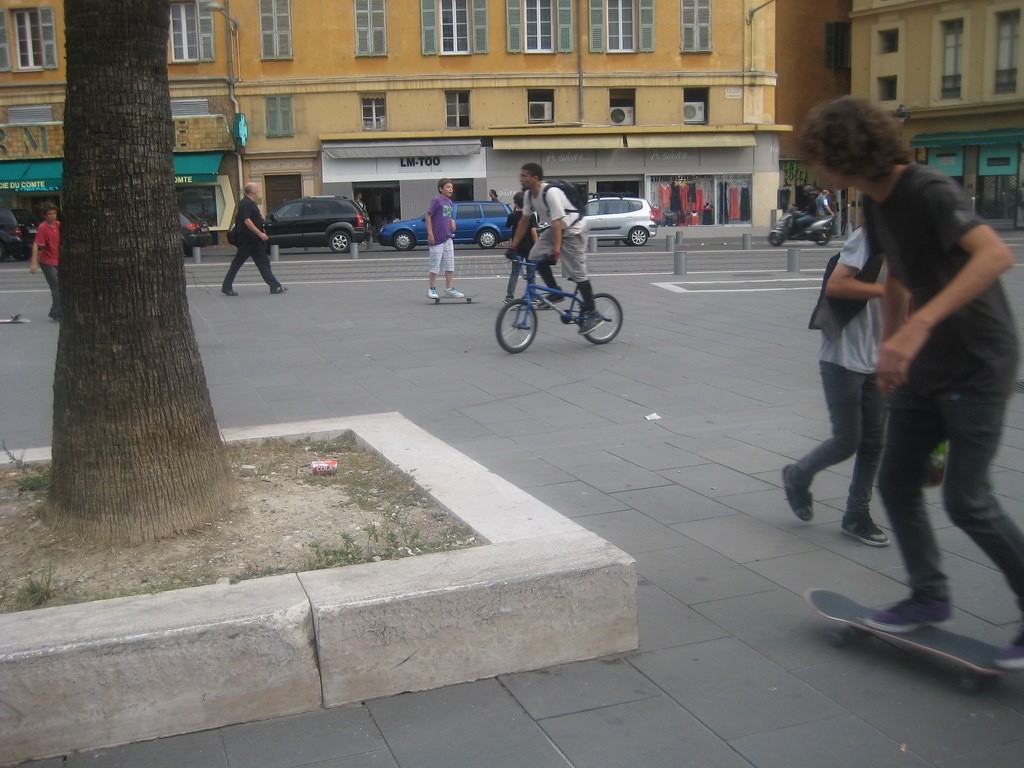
[0,206,43,263]
[179,210,212,257]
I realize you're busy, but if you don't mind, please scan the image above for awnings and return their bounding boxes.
[909,128,1024,147]
[173,152,223,186]
[323,138,482,158]
[0,160,64,195]
[492,134,757,149]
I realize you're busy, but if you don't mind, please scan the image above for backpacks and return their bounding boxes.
[529,179,588,225]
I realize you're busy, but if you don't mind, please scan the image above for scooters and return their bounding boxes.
[767,203,836,247]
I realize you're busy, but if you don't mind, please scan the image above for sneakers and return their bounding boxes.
[578,310,605,335]
[428,287,438,298]
[443,287,464,298]
[782,464,814,521]
[993,640,1024,669]
[840,512,890,546]
[221,291,238,296]
[270,286,289,294]
[864,597,953,633]
[537,286,565,309]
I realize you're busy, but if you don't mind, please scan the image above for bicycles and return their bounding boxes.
[494,252,623,354]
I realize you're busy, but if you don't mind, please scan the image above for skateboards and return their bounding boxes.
[802,587,1024,694]
[0,312,32,323]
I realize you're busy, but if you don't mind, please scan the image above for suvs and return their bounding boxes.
[375,200,514,253]
[226,195,371,255]
[541,191,659,247]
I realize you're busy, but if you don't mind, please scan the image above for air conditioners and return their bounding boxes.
[683,101,704,121]
[610,106,634,126]
[528,101,552,121]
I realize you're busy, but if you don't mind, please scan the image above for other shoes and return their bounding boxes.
[48,314,60,321]
[504,298,514,302]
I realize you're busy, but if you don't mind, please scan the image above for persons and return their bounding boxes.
[489,189,499,201]
[790,185,836,238]
[355,191,368,244]
[782,225,890,547]
[30,201,61,321]
[425,178,464,299]
[799,95,1024,671]
[222,182,288,296]
[499,162,604,335]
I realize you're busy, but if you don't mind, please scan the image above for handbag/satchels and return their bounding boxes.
[227,225,238,247]
